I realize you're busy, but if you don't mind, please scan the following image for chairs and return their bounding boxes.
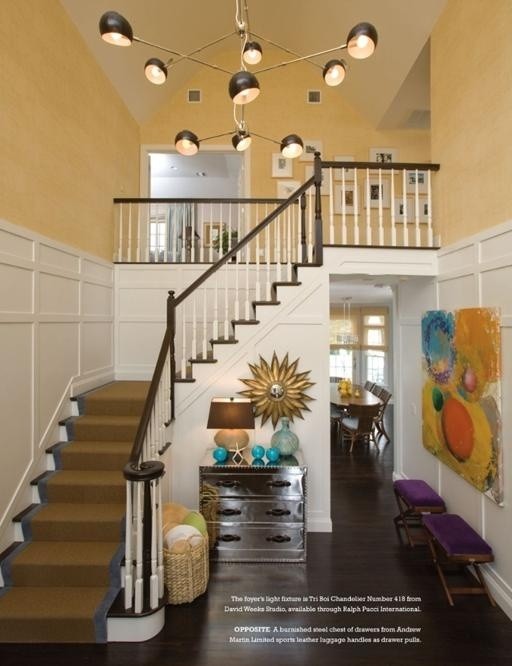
[365,381,375,391]
[371,385,384,397]
[330,404,342,435]
[331,375,342,383]
[341,404,382,453]
[375,390,392,443]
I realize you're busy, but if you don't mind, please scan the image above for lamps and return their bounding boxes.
[207,398,256,460]
[179,225,200,262]
[335,297,359,354]
[99,0,377,159]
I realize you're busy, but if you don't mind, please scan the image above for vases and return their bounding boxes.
[270,417,299,455]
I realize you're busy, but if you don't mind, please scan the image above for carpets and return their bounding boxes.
[0,380,154,642]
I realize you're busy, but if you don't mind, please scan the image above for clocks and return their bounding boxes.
[235,351,316,431]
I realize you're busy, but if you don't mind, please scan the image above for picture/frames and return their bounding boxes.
[405,169,428,194]
[334,184,360,214]
[300,138,322,161]
[272,152,294,179]
[421,308,504,508]
[367,147,398,174]
[394,195,414,224]
[333,154,356,181]
[305,164,328,195]
[204,222,226,248]
[417,196,429,224]
[278,181,300,199]
[365,177,390,208]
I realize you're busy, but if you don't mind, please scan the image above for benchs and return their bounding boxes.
[422,514,494,609]
[394,479,446,547]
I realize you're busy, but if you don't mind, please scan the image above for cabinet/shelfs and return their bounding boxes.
[197,445,309,563]
